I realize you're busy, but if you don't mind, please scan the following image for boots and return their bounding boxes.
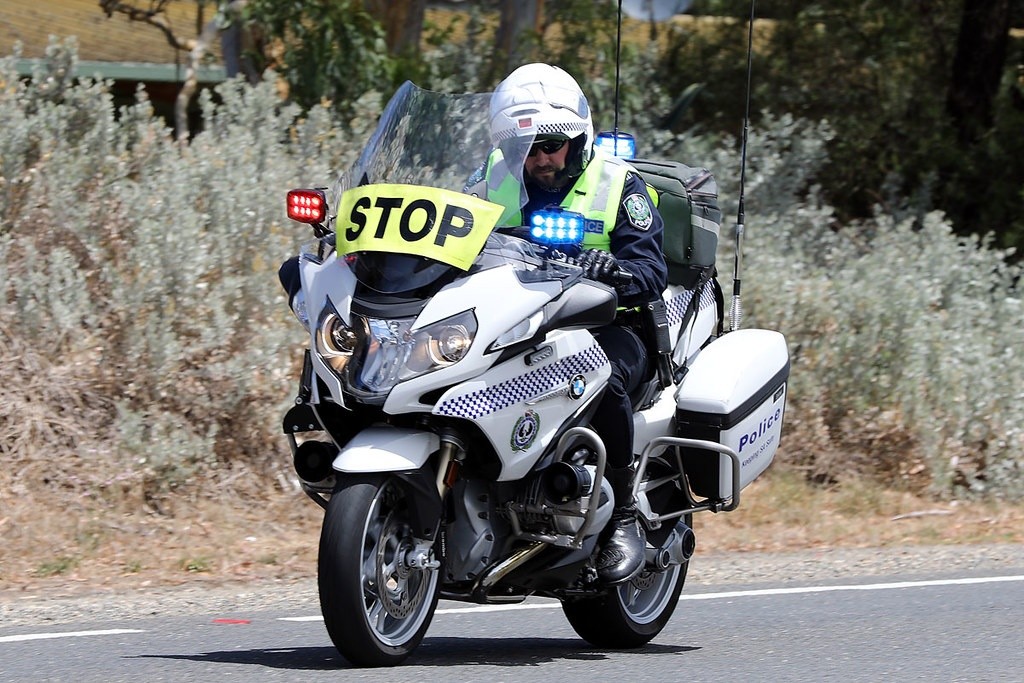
[599,454,646,585]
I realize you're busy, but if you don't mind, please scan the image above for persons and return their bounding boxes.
[463,63,669,587]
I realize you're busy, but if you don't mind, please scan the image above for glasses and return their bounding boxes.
[515,138,566,156]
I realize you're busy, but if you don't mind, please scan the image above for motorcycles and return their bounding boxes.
[278,80,789,667]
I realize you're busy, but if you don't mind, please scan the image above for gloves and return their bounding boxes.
[573,247,631,287]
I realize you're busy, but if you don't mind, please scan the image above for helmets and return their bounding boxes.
[488,62,595,178]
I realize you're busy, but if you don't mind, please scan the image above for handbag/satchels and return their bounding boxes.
[621,155,722,270]
